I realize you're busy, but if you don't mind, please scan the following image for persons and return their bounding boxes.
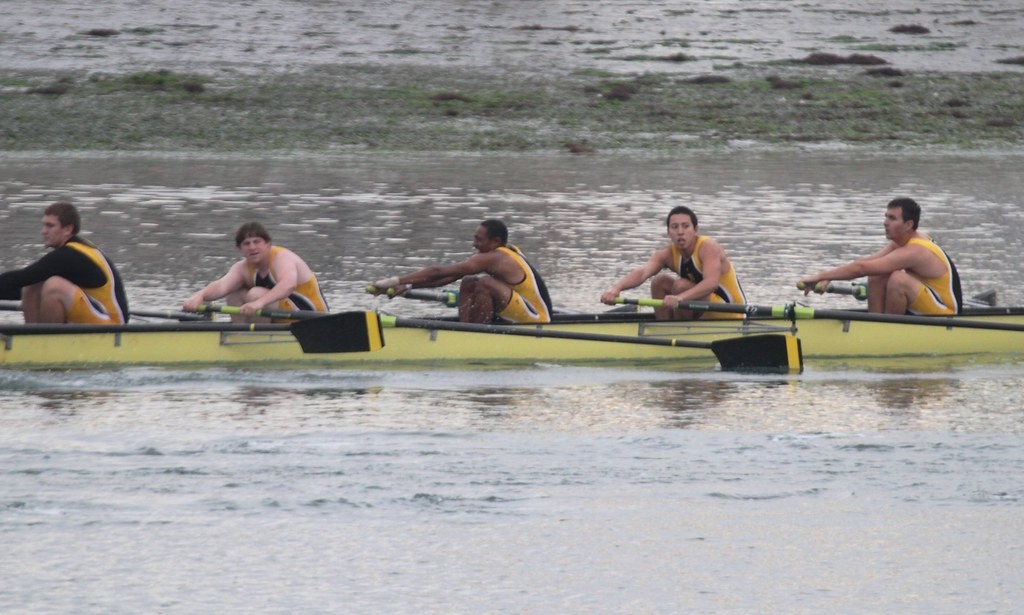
[368,220,552,325]
[798,198,963,315]
[601,206,748,320]
[182,221,329,323]
[0,203,129,324]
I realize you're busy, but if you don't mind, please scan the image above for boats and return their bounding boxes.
[0,307,1023,371]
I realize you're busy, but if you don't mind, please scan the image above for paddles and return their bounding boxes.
[796,281,998,308]
[196,303,805,377]
[0,309,387,355]
[600,296,1024,332]
[364,284,641,315]
[0,299,216,326]
